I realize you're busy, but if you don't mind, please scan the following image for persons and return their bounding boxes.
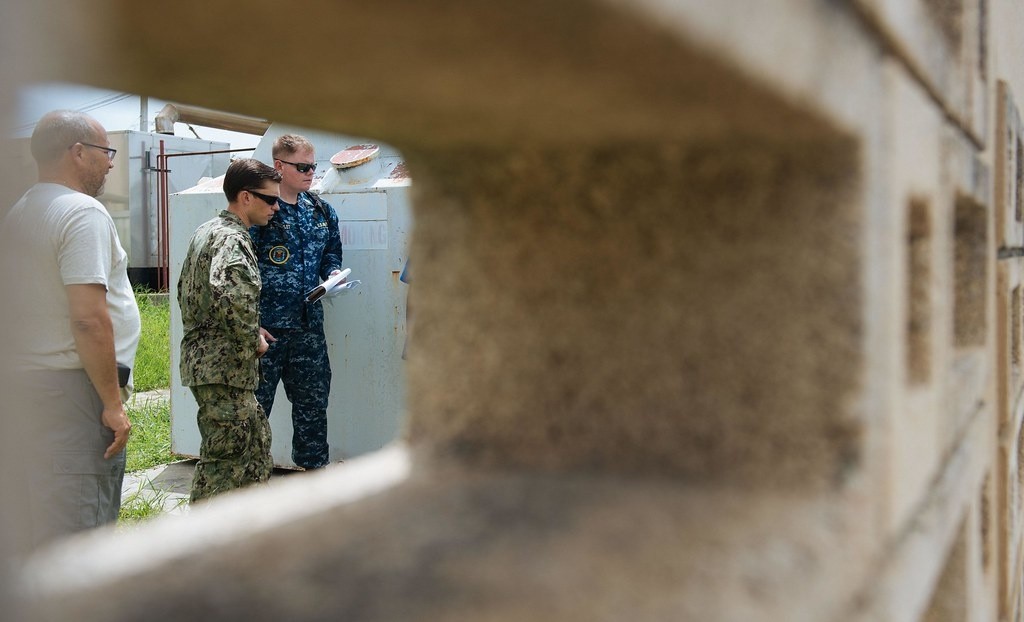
[250,135,343,476]
[177,158,282,504]
[1,109,141,548]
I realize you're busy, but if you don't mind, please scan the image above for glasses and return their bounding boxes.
[239,188,279,206]
[273,158,317,173]
[68,142,117,160]
